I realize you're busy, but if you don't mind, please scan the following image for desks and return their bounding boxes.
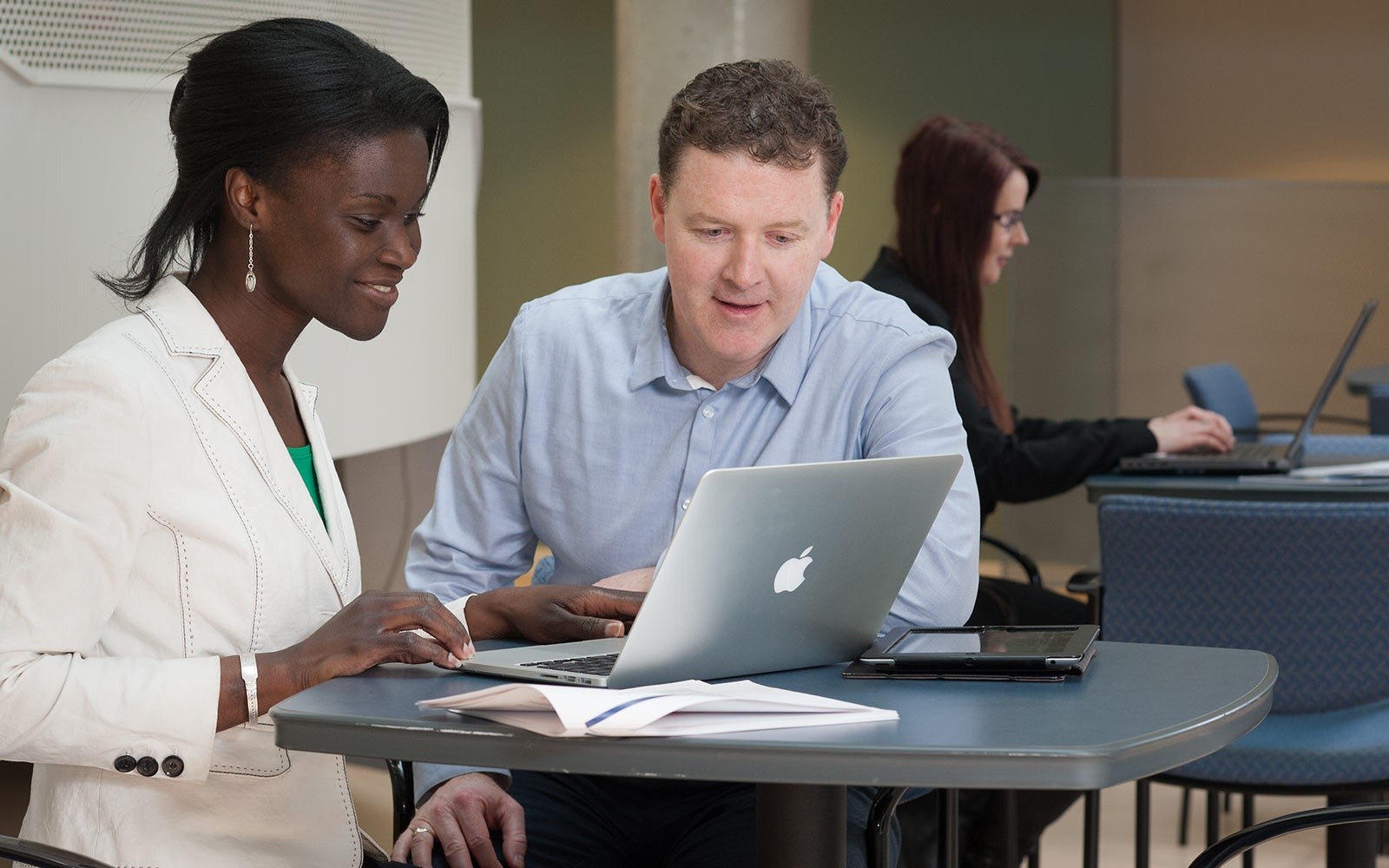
[1087,453,1389,504]
[274,626,1279,867]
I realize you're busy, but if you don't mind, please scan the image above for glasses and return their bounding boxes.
[993,210,1021,228]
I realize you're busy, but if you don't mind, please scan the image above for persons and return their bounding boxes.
[864,116,1236,868]
[0,19,648,868]
[390,58,980,868]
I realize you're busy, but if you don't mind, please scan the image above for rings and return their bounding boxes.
[412,827,435,839]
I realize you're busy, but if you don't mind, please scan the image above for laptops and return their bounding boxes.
[1121,299,1379,471]
[433,452,964,690]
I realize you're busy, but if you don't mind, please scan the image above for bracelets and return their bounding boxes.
[239,652,259,724]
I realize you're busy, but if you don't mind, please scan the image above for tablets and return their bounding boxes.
[858,624,1102,673]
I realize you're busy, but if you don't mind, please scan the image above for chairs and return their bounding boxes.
[922,364,1389,868]
